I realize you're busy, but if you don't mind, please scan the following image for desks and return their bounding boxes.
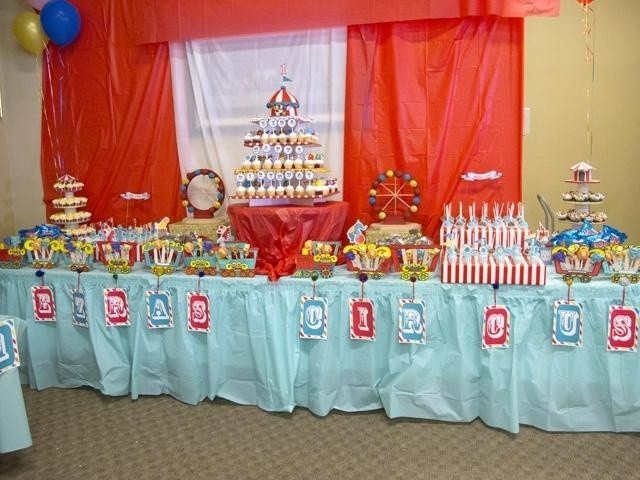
[280,265,640,435]
[227,201,349,275]
[0,263,298,414]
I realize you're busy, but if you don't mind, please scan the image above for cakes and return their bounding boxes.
[49,182,92,223]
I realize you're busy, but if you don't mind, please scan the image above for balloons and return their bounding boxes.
[40,0,81,47]
[26,0,49,11]
[13,13,50,55]
